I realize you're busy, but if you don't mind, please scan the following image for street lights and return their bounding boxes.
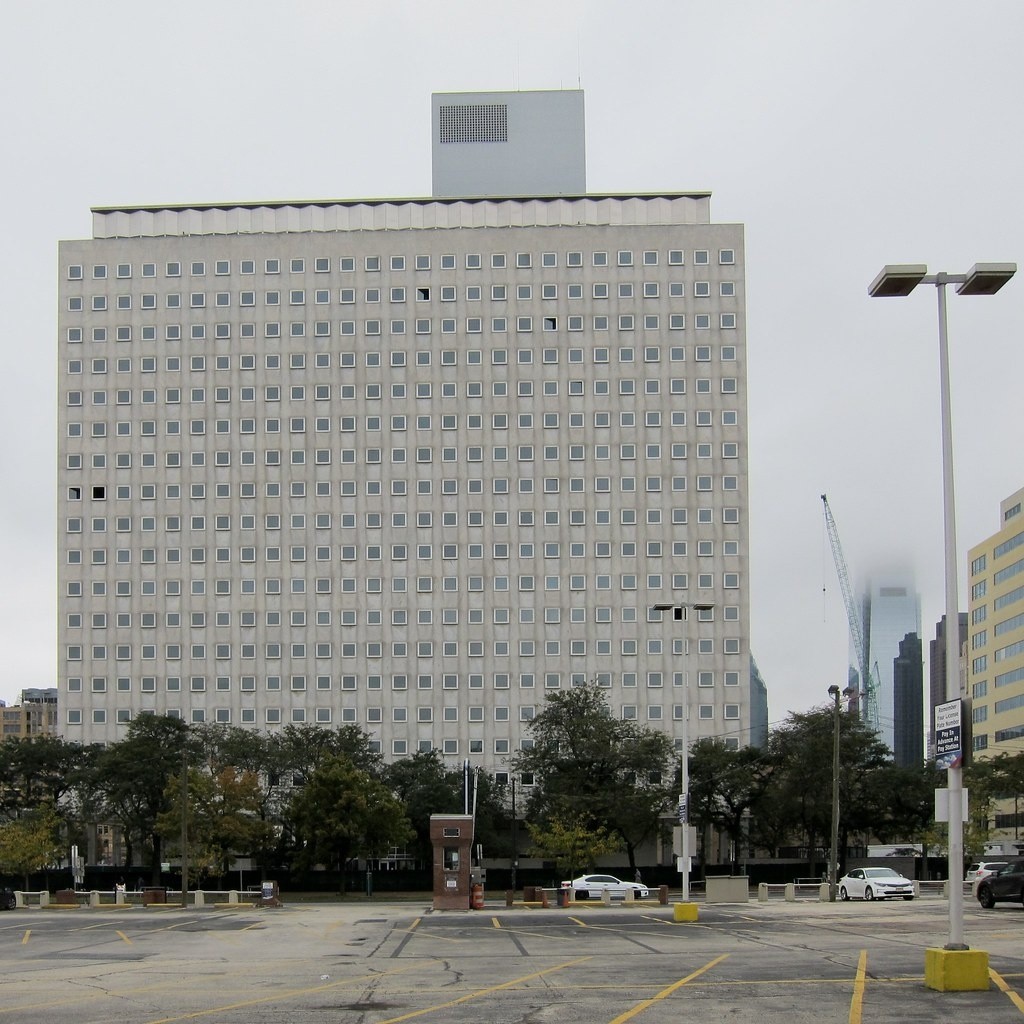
[649,600,719,921]
[826,685,857,904]
[865,259,1017,996]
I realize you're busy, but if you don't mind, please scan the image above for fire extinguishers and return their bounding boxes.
[473,883,483,908]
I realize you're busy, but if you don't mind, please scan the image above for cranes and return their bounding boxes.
[816,491,884,739]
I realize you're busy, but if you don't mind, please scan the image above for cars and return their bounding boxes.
[977,855,1024,914]
[966,861,1015,885]
[839,865,915,901]
[561,874,648,903]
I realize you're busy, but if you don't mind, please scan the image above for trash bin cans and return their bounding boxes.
[524,886,542,901]
[143,886,167,906]
[557,888,570,907]
